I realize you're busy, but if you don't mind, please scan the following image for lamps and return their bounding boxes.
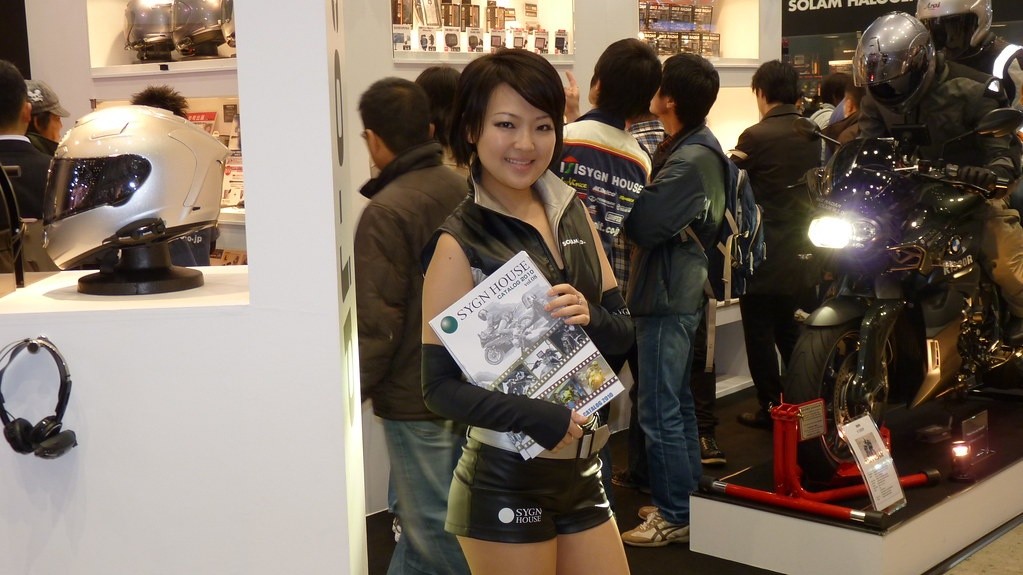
[948,438,977,485]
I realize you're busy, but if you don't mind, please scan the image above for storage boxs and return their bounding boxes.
[640,3,721,56]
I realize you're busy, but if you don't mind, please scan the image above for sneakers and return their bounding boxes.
[637,505,661,520]
[622,513,690,547]
[611,471,650,491]
[699,437,727,464]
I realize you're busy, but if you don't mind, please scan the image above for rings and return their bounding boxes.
[577,295,583,305]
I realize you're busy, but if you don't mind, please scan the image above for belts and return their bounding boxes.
[467,412,612,461]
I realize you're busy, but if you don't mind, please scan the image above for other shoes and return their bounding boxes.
[735,411,772,429]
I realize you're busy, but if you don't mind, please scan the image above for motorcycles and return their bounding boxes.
[512,328,550,355]
[477,312,536,365]
[783,108,1023,480]
[523,338,562,379]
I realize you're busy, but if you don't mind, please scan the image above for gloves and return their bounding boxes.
[956,164,999,199]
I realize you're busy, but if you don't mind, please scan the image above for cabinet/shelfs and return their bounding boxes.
[85,0,250,251]
[634,0,762,400]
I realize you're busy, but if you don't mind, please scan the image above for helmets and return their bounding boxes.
[913,0,993,52]
[40,104,227,271]
[851,12,937,115]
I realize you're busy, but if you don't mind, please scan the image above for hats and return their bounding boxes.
[23,79,72,117]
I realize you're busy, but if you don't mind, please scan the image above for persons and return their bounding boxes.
[0,60,55,274]
[622,92,666,160]
[353,77,471,575]
[124,84,221,267]
[546,38,662,503]
[799,9,1023,342]
[914,0,1023,145]
[415,65,471,178]
[24,80,71,158]
[729,60,867,423]
[621,52,734,546]
[419,47,632,575]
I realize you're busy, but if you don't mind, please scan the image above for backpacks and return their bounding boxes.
[669,132,767,303]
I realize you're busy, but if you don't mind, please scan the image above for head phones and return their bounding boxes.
[0,338,78,460]
